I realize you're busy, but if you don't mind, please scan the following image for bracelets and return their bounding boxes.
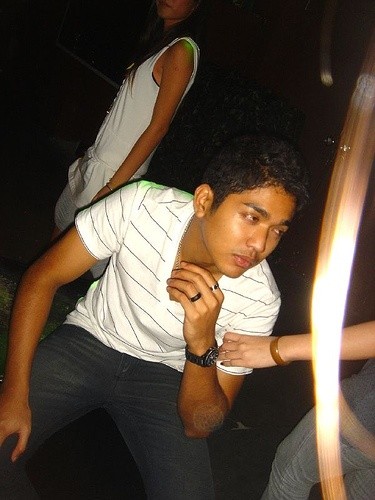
[104,181,117,193]
[269,334,290,366]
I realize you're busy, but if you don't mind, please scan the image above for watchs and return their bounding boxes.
[184,339,219,367]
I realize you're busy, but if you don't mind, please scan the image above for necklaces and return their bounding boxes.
[176,211,198,271]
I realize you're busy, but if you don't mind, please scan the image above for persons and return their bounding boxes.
[0,134,311,500]
[51,0,201,293]
[215,318,375,500]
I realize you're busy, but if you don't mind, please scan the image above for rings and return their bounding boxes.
[188,291,201,302]
[210,280,219,292]
